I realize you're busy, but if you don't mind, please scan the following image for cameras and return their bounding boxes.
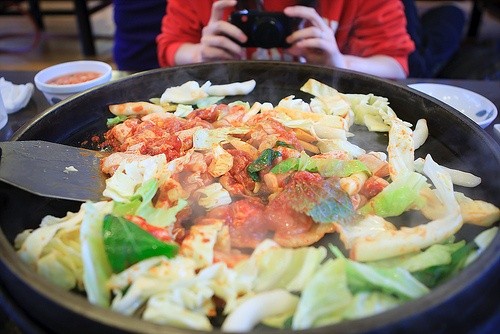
[230,9,298,49]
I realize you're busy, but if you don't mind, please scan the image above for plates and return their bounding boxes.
[408,83,498,130]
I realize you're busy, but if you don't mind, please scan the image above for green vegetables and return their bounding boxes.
[102,201,180,273]
[344,241,479,300]
[248,140,429,227]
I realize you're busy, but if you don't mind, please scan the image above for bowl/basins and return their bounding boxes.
[34,60,112,106]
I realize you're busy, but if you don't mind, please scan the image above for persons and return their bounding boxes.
[156,0,415,79]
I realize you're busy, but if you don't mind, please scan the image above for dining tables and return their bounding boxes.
[1,69,500,334]
